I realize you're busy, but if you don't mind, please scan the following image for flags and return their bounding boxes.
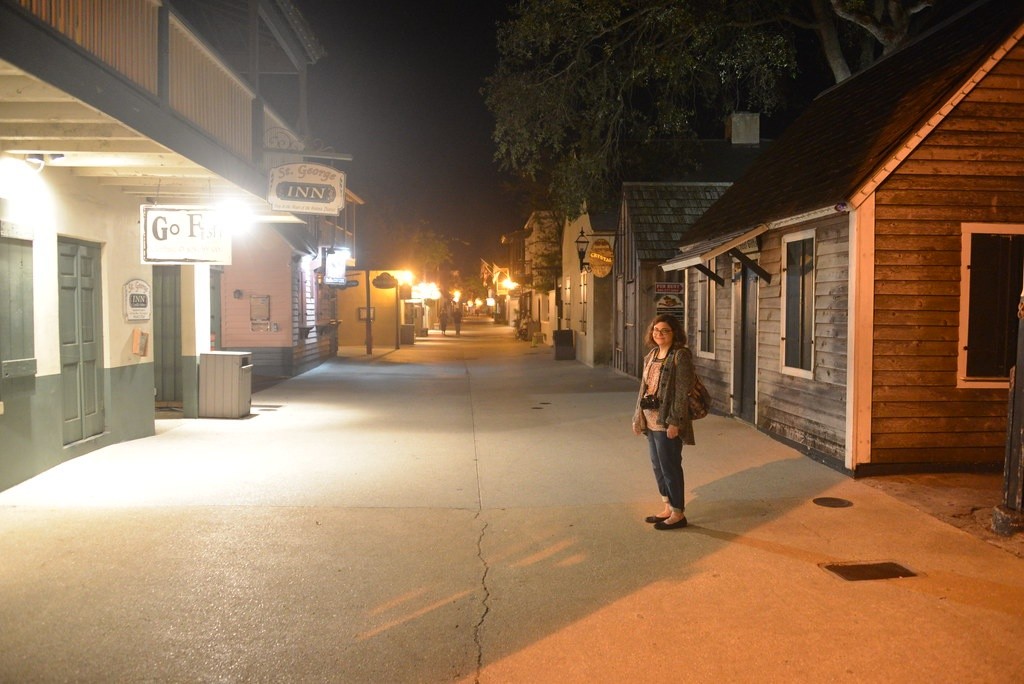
[480,262,500,287]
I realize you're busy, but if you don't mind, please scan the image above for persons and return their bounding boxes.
[451,308,463,335]
[438,309,448,334]
[631,315,695,531]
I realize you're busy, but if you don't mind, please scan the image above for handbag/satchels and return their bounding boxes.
[675,349,710,420]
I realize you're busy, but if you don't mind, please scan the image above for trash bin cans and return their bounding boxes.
[552,330,574,360]
[400,324,414,344]
[199,350,253,418]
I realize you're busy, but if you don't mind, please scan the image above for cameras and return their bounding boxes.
[640,394,659,409]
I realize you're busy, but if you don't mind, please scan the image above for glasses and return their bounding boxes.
[651,327,674,335]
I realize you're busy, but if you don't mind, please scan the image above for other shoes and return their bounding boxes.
[645,514,687,530]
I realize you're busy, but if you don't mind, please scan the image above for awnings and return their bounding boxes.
[658,224,775,285]
